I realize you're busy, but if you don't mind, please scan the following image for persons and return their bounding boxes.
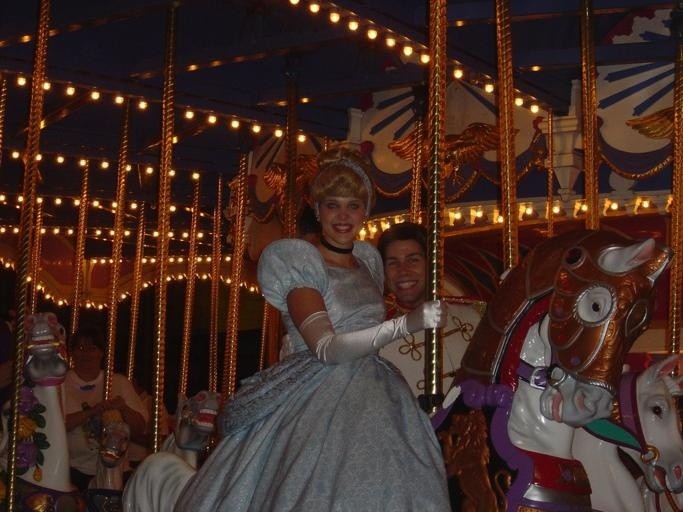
[375,222,430,319]
[61,329,149,490]
[173,157,453,512]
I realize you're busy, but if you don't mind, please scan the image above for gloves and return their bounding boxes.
[299,299,448,366]
